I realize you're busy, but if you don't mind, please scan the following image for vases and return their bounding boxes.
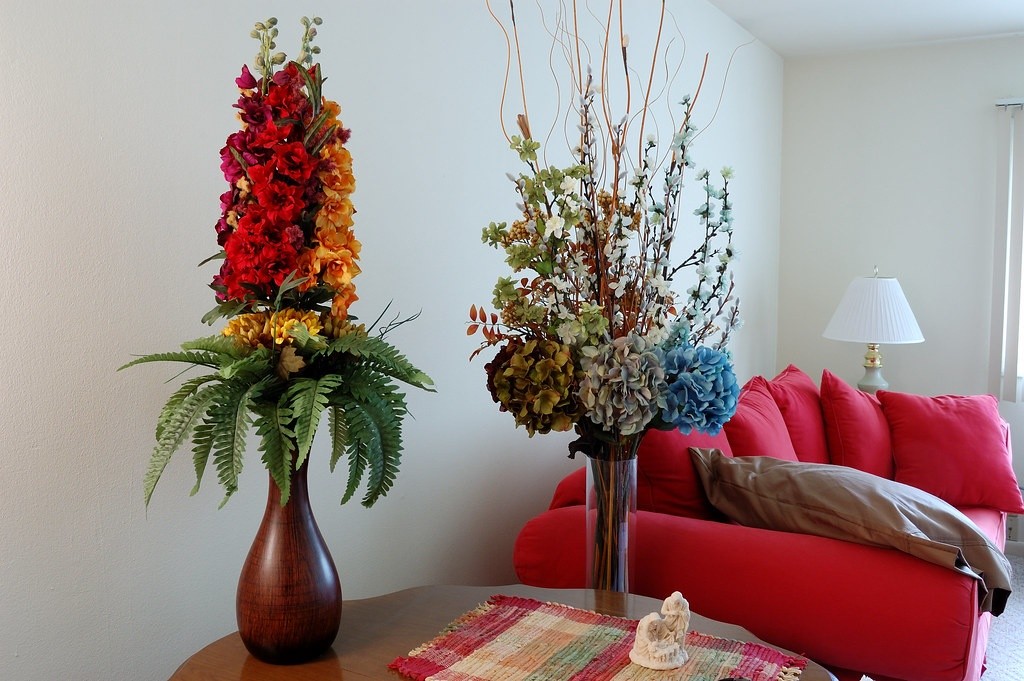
[237,421,344,668]
[583,456,638,622]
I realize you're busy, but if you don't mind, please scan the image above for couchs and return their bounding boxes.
[512,387,1013,681]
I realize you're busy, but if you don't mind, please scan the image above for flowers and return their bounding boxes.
[465,0,745,593]
[113,12,455,515]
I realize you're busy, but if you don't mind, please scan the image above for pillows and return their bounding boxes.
[766,365,830,465]
[688,444,1013,619]
[876,389,1024,516]
[820,370,895,479]
[722,375,800,462]
[637,426,736,520]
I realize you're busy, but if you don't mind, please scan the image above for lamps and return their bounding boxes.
[823,265,926,394]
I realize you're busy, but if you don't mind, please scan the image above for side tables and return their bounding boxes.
[168,581,840,681]
